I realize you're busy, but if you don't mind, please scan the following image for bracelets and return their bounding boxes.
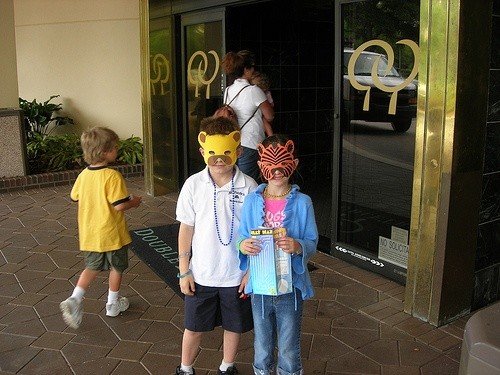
[176,270,192,278]
[179,251,192,258]
[292,250,302,256]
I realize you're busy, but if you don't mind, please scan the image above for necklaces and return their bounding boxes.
[210,174,235,246]
[265,186,292,197]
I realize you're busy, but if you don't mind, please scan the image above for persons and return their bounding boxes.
[222,50,274,184]
[175,116,258,375]
[235,134,318,375]
[59,127,143,329]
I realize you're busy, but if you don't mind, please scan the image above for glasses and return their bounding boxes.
[247,65,258,71]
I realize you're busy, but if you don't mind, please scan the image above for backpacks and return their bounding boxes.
[212,84,259,135]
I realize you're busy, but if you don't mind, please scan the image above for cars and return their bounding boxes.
[343,49,419,132]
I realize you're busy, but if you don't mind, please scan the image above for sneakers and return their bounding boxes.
[59,296,83,330]
[105,296,129,317]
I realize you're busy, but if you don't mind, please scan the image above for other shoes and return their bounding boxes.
[216,365,238,375]
[175,365,196,375]
